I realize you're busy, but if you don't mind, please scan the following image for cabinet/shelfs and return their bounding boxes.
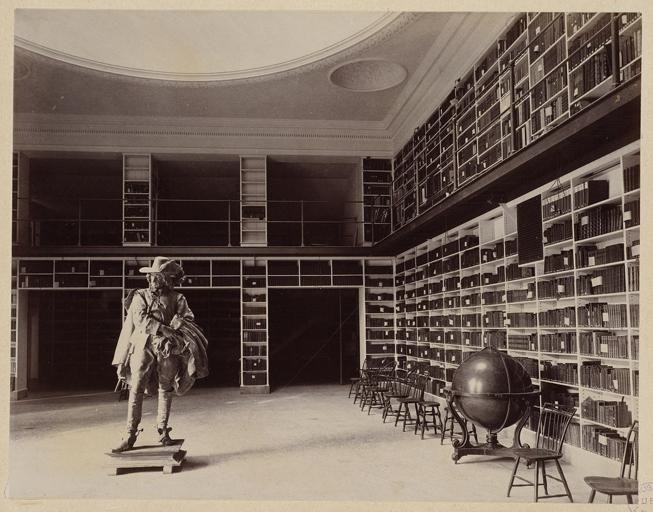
[364,14,639,482]
[13,155,362,392]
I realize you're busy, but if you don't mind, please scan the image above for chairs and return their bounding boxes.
[347,363,635,502]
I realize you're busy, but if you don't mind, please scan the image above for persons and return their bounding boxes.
[110,258,210,453]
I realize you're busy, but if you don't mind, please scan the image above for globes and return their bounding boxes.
[439,345,542,469]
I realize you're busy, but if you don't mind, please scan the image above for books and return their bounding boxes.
[241,258,268,386]
